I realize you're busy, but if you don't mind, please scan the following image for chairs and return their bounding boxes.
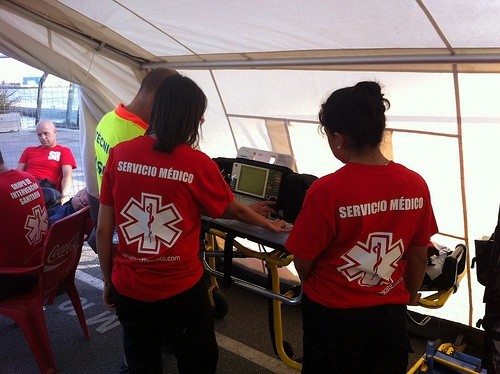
[0,205,92,374]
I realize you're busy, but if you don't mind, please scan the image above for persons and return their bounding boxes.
[88,67,276,257]
[95,75,292,374]
[16,119,77,211]
[48,187,88,225]
[284,81,439,374]
[0,150,48,303]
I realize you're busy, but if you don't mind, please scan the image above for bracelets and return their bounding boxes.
[102,279,111,287]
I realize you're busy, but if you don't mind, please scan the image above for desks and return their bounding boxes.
[198,215,302,371]
